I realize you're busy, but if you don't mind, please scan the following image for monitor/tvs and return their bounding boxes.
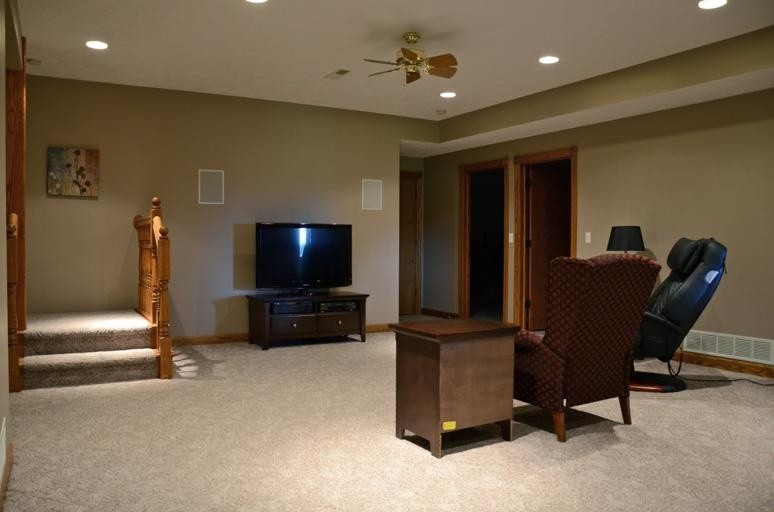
[255,222,353,296]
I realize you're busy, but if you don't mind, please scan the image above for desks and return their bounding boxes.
[388,319,521,458]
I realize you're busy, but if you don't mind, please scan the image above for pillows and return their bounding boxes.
[667,238,705,277]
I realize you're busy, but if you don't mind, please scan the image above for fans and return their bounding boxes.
[364,32,457,84]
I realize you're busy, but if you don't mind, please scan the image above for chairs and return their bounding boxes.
[629,238,726,393]
[515,253,662,443]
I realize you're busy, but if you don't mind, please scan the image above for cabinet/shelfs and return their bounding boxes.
[244,292,369,350]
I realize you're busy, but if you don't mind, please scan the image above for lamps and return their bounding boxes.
[606,226,646,253]
